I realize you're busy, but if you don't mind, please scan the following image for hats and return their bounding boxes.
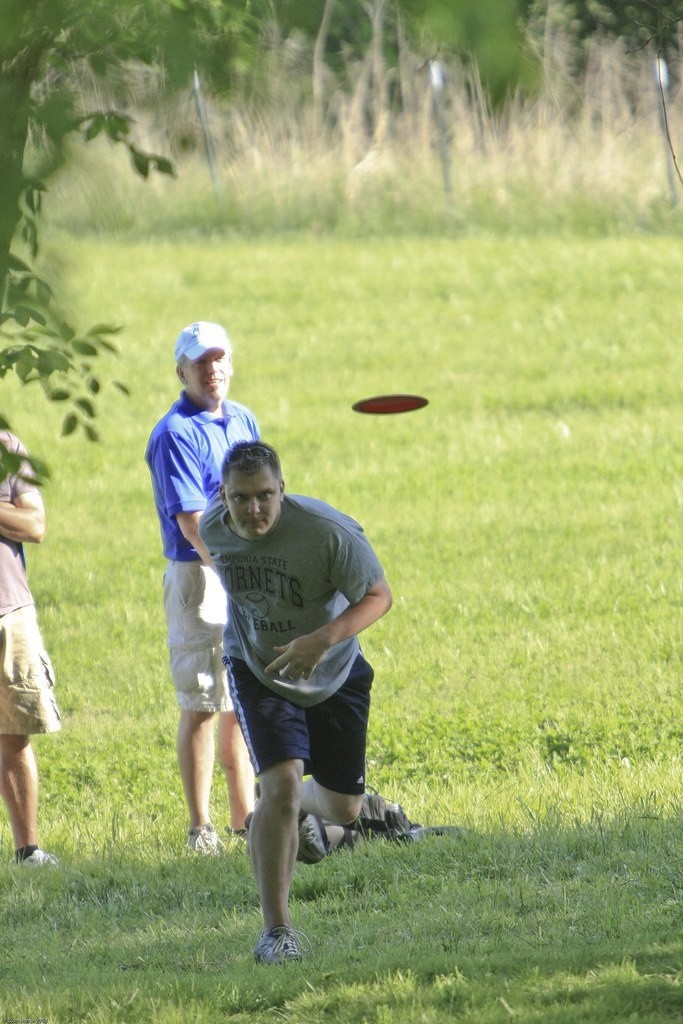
[175,321,233,363]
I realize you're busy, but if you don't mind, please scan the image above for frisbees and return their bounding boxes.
[352,395,428,414]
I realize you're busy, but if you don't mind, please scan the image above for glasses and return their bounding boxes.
[226,447,278,464]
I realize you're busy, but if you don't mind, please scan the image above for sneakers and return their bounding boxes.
[18,850,61,874]
[296,816,325,864]
[254,926,312,968]
[189,827,221,857]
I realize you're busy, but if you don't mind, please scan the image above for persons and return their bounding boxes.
[0,429,63,868]
[198,439,392,966]
[144,321,259,854]
